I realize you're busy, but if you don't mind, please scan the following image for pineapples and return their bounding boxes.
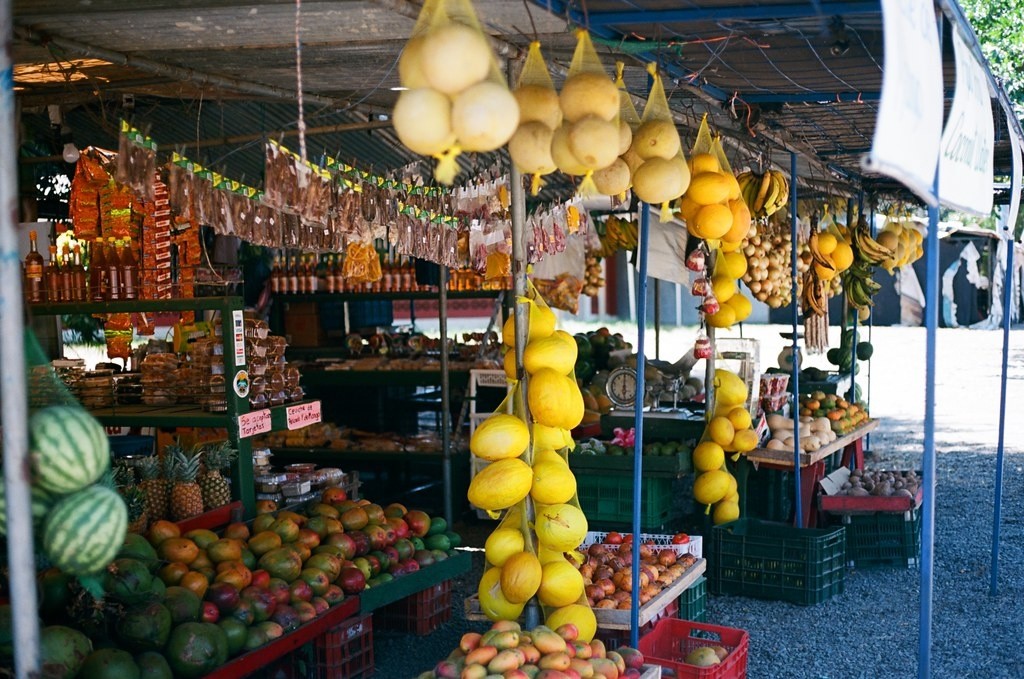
[100,441,237,533]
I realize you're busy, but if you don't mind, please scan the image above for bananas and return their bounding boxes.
[606,216,637,251]
[843,214,893,311]
[737,169,788,219]
[804,229,836,315]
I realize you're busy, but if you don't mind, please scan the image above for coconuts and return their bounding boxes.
[0,530,228,679]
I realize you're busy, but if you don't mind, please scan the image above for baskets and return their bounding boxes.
[575,532,703,561]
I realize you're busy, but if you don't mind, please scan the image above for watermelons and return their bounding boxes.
[0,471,51,536]
[28,407,109,494]
[43,485,127,574]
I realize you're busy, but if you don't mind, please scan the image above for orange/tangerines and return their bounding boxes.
[572,441,691,455]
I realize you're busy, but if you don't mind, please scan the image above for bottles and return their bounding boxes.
[122,236,137,299]
[47,245,59,301]
[60,246,73,300]
[25,230,44,303]
[91,238,106,300]
[449,263,513,291]
[105,237,123,299]
[73,245,86,300]
[271,250,432,293]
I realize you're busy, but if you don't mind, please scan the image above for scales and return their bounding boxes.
[605,369,695,419]
[776,331,806,372]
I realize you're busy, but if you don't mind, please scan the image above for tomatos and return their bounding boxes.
[604,532,690,544]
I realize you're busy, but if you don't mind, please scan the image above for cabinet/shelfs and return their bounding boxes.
[0,245,881,678]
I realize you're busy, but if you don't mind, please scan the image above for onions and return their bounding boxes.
[580,543,696,609]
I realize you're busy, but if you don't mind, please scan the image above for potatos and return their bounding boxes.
[834,468,920,497]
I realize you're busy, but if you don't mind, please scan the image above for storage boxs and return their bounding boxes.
[708,519,848,606]
[261,611,373,679]
[580,531,703,558]
[576,477,676,529]
[567,433,692,480]
[677,575,708,639]
[592,598,679,649]
[158,425,229,451]
[372,579,452,637]
[320,296,392,330]
[841,500,923,568]
[285,299,345,347]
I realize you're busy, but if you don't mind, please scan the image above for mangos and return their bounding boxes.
[147,493,461,653]
[420,619,644,679]
[792,389,871,437]
[577,327,633,410]
